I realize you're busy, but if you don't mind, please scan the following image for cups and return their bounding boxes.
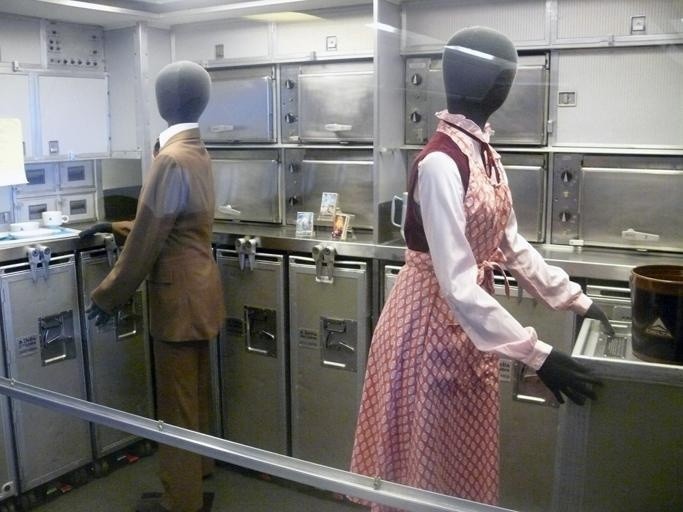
[42,211,68,227]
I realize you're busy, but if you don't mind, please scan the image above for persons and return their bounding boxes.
[342,25,614,512]
[78,61,227,512]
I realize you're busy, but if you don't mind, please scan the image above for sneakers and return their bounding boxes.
[141,489,215,511]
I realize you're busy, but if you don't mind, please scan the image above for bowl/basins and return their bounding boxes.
[10,222,40,231]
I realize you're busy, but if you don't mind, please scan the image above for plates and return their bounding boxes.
[10,229,62,239]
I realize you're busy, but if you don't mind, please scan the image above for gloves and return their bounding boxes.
[576,304,615,342]
[79,222,112,239]
[86,298,110,328]
[536,346,604,406]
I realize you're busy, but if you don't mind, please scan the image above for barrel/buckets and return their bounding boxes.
[627,266,683,367]
